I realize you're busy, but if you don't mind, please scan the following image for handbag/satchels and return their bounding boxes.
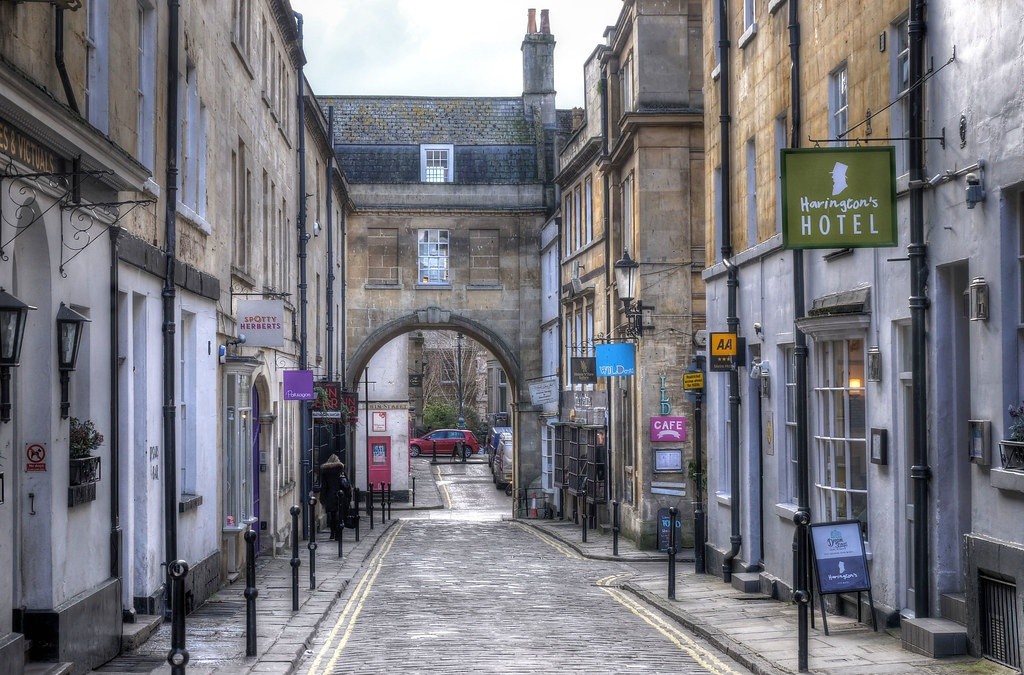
[343,507,360,529]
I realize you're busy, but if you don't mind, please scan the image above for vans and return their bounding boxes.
[488,426,513,490]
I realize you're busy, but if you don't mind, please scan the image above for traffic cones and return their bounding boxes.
[528,492,539,519]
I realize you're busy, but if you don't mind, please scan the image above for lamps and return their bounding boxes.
[613,249,640,317]
[0,284,38,424]
[55,300,92,420]
[849,379,860,400]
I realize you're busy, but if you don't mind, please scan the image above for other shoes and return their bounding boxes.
[329,534,334,539]
[335,537,339,541]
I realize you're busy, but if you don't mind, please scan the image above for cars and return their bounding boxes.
[409,428,480,458]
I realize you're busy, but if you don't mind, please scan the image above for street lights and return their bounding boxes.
[456,333,466,430]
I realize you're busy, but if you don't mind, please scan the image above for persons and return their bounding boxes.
[319,455,353,544]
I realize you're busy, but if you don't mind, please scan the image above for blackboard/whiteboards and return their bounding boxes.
[657,507,681,552]
